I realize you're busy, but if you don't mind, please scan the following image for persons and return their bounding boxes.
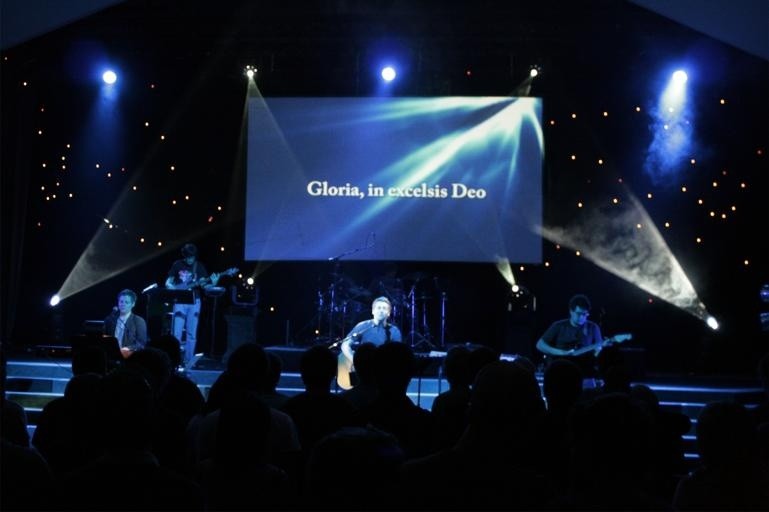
[165,242,220,370]
[102,289,147,352]
[342,296,401,363]
[536,295,603,364]
[0,337,769,511]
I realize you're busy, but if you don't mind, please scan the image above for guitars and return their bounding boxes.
[173,267,240,291]
[544,333,631,372]
[336,349,447,391]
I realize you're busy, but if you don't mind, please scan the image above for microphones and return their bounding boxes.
[143,283,158,293]
[384,323,392,338]
